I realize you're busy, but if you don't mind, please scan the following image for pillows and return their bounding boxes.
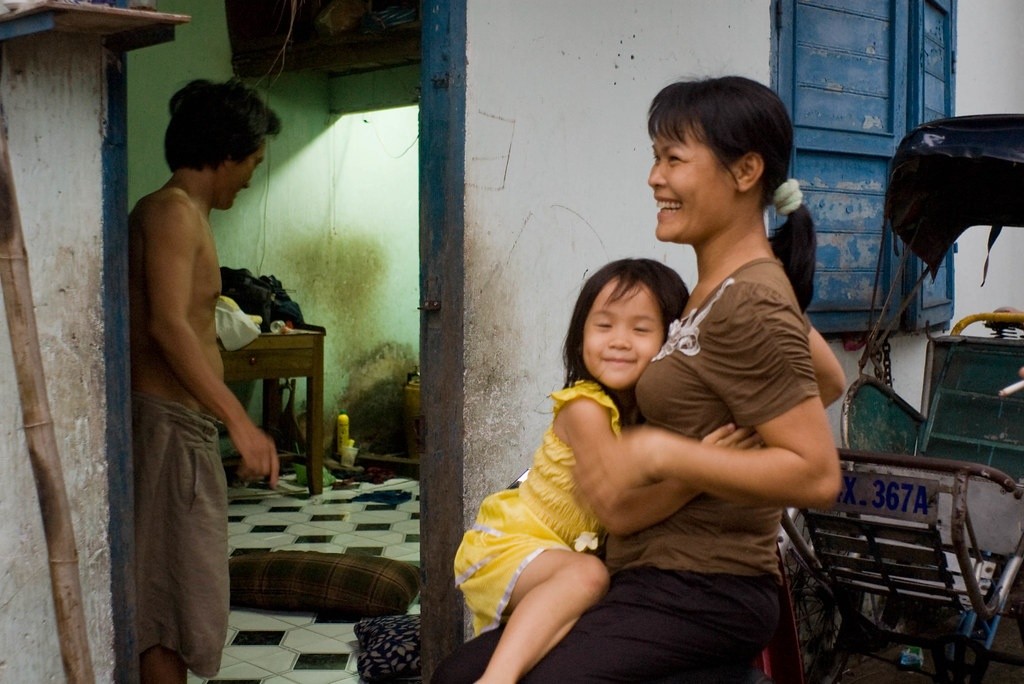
[229,552,420,616]
[353,614,422,682]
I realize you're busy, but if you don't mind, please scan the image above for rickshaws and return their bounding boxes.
[770,110,1024,684]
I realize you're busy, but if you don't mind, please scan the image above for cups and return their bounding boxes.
[340,445,359,468]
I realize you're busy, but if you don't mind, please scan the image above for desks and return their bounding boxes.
[216,333,323,495]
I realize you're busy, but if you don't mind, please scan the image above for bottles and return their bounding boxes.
[337,408,349,456]
[270,320,286,333]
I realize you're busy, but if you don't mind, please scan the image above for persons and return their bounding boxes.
[128,78,280,684]
[452,258,766,684]
[430,75,841,683]
